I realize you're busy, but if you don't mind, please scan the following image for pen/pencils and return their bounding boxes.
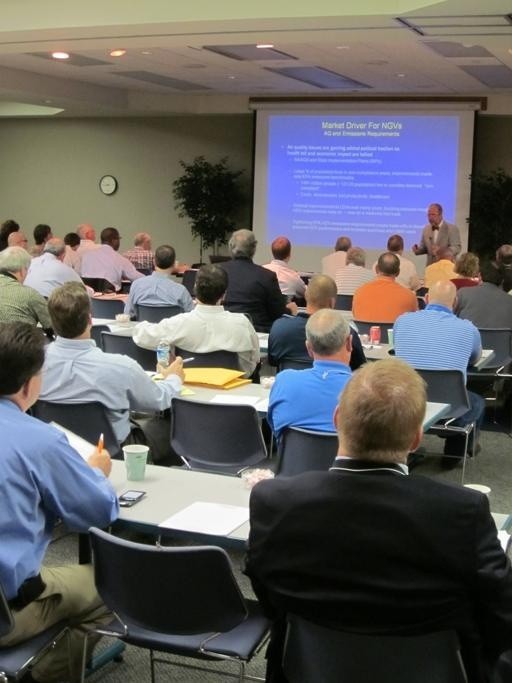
[182,357,195,363]
[98,432,104,453]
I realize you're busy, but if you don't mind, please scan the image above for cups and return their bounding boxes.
[241,468,276,491]
[358,326,394,346]
[121,443,149,482]
[116,313,131,327]
[463,483,490,500]
[259,374,275,389]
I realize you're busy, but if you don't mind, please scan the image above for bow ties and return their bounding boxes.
[433,225,439,230]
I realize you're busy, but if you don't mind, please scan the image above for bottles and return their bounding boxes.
[156,336,171,374]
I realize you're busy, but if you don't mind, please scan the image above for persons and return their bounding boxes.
[420,244,511,366]
[372,234,420,291]
[413,204,462,266]
[321,236,353,276]
[333,247,376,297]
[218,228,298,333]
[37,280,186,464]
[0,219,156,300]
[262,236,306,299]
[266,308,354,444]
[268,274,368,370]
[0,246,52,335]
[392,279,486,469]
[1,319,119,669]
[131,264,260,379]
[124,245,194,318]
[247,356,512,683]
[352,253,421,324]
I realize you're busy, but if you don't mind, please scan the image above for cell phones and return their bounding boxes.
[118,490,146,507]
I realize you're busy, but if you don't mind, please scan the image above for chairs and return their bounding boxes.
[26,399,120,460]
[274,425,339,477]
[169,397,275,479]
[1,256,510,426]
[411,367,476,487]
[80,524,271,681]
[281,612,465,681]
[0,623,73,681]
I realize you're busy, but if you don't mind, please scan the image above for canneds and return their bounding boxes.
[369,326,381,346]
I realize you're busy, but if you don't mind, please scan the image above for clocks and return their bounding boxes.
[98,173,119,195]
